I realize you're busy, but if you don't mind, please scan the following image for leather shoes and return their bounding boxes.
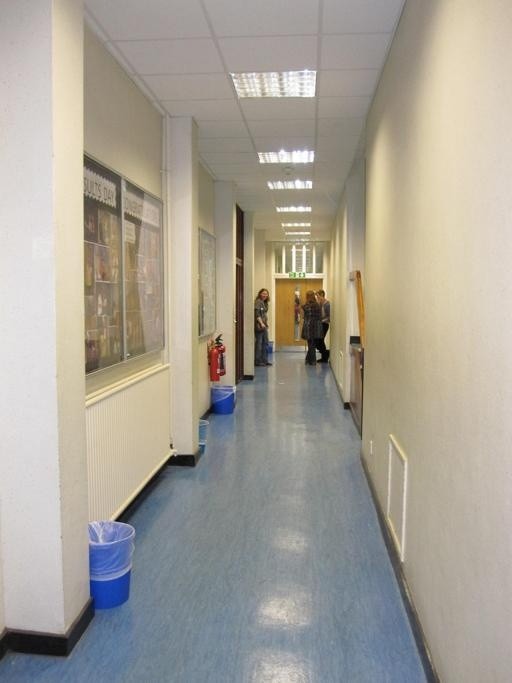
[265,362,272,365]
[317,358,328,363]
[255,362,265,366]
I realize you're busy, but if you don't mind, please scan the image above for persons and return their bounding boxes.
[255,288,271,366]
[295,298,301,339]
[301,290,325,365]
[315,291,330,363]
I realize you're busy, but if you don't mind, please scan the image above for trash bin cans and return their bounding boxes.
[88,521,136,609]
[199,420,209,453]
[266,341,273,353]
[211,385,236,414]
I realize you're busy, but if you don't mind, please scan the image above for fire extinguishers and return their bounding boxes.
[208,334,226,382]
